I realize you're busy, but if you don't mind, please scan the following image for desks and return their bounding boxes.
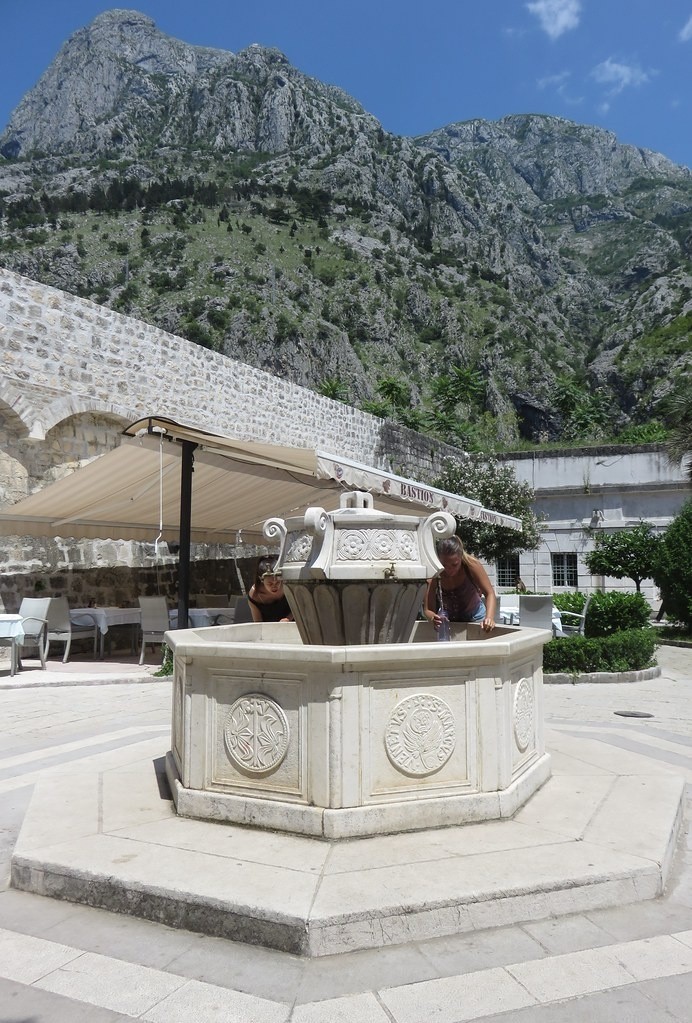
[0,613,25,677]
[168,607,235,630]
[500,607,562,632]
[68,607,141,661]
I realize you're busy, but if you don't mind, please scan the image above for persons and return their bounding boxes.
[423,535,497,632]
[248,555,295,622]
[514,577,526,592]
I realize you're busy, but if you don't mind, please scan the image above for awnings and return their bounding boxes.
[0,415,523,629]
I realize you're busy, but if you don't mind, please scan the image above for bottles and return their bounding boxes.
[438,611,450,642]
[89,598,97,609]
[167,597,178,609]
[122,599,139,608]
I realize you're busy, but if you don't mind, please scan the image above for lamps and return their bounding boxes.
[592,510,608,521]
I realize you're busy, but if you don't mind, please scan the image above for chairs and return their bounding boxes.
[137,595,194,666]
[43,597,98,663]
[0,597,52,675]
[197,594,254,624]
[479,595,591,639]
